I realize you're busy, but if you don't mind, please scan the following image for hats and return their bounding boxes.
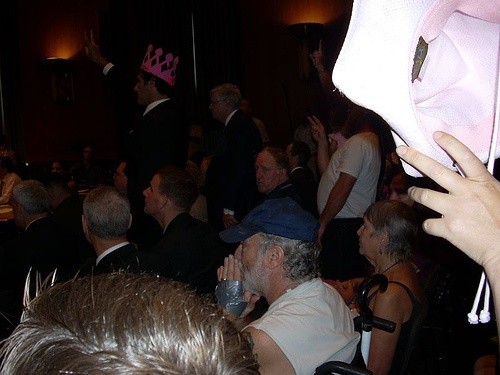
[219,197,320,244]
[332,0,498,177]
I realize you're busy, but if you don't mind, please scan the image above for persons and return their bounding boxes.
[213,196,363,375]
[5,179,56,234]
[0,37,500,374]
[206,84,265,226]
[81,28,189,258]
[142,167,234,296]
[322,199,427,375]
[0,265,266,375]
[80,184,142,275]
[395,130,500,343]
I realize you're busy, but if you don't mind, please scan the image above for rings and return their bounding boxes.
[220,277,227,281]
